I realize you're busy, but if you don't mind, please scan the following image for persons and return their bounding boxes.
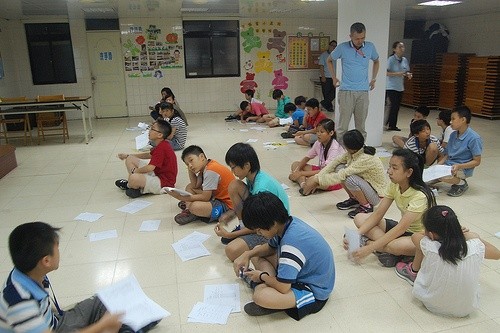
[165,93,188,126]
[224,85,330,145]
[149,86,180,120]
[215,142,291,262]
[288,118,347,196]
[389,105,430,149]
[386,40,413,131]
[0,221,160,333]
[435,104,483,196]
[303,129,390,218]
[115,118,178,198]
[163,144,236,224]
[402,118,445,191]
[318,39,337,112]
[233,190,335,320]
[395,204,500,316]
[148,101,188,150]
[436,109,456,156]
[326,22,380,148]
[342,148,437,267]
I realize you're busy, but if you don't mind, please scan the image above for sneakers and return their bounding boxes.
[225,116,237,121]
[126,187,148,198]
[394,262,418,286]
[336,197,360,209]
[221,225,249,245]
[344,234,369,247]
[447,178,468,197]
[348,204,373,218]
[378,253,408,267]
[175,209,199,224]
[115,179,129,190]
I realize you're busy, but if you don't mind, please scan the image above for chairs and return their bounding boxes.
[37,94,69,145]
[0,97,33,146]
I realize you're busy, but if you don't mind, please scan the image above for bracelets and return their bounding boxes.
[127,153,129,158]
[131,167,135,173]
[259,271,269,282]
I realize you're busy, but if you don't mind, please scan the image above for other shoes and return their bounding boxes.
[281,133,295,138]
[243,301,285,316]
[388,127,401,130]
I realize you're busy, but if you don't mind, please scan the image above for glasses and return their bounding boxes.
[231,163,237,171]
[150,127,164,134]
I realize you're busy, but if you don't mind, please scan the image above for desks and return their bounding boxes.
[0,95,93,145]
